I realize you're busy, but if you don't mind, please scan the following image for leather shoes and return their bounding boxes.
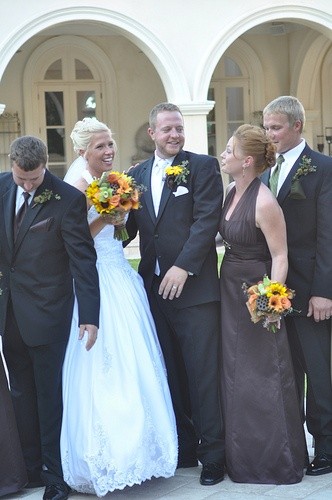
[200,462,224,485]
[176,459,199,468]
[43,484,70,500]
[305,455,332,475]
[25,480,45,488]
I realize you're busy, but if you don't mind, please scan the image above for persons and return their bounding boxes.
[0,136,100,500]
[217,124,310,484]
[58,117,177,497]
[258,95,332,475]
[122,104,224,485]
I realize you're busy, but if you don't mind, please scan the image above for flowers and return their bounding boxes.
[32,189,60,209]
[241,274,303,334]
[165,160,191,185]
[290,155,318,182]
[85,171,147,241]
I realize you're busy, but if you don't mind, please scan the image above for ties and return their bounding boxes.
[16,192,31,235]
[268,154,285,197]
[157,159,170,174]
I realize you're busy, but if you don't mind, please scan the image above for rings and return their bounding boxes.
[173,286,176,288]
